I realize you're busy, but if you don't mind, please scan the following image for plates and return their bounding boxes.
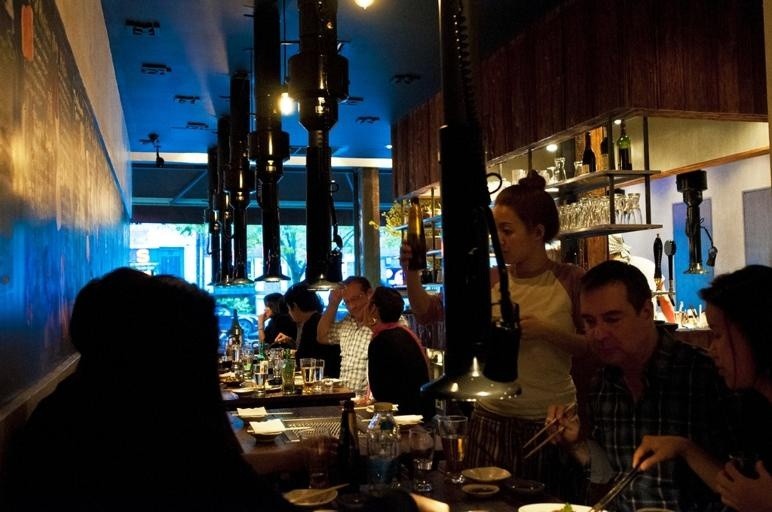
[393,113,662,393]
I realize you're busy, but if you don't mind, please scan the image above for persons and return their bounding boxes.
[273,323,303,350]
[399,169,590,484]
[544,259,722,512]
[27,275,347,512]
[253,289,285,348]
[630,264,770,511]
[70,266,340,482]
[362,285,429,418]
[279,286,323,364]
[316,276,372,404]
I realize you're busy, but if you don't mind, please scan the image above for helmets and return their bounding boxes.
[517,502,611,512]
[246,430,281,443]
[461,484,499,496]
[461,467,511,482]
[233,414,265,425]
[504,479,545,494]
[365,406,399,416]
[400,423,415,433]
[283,489,338,506]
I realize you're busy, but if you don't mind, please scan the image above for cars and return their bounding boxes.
[438,415,468,482]
[407,428,435,491]
[300,429,332,487]
[216,342,343,393]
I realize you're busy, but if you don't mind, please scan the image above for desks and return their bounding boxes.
[219,342,608,511]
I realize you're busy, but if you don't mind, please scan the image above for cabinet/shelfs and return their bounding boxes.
[281,348,296,394]
[366,402,401,461]
[258,341,266,361]
[228,308,242,347]
[339,400,361,461]
[393,113,662,393]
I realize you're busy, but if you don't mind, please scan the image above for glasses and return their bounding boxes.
[675,167,718,276]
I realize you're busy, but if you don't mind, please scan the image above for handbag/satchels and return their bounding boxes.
[551,326,562,344]
[257,326,264,332]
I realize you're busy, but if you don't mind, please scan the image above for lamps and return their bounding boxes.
[202,1,526,405]
[675,167,718,276]
